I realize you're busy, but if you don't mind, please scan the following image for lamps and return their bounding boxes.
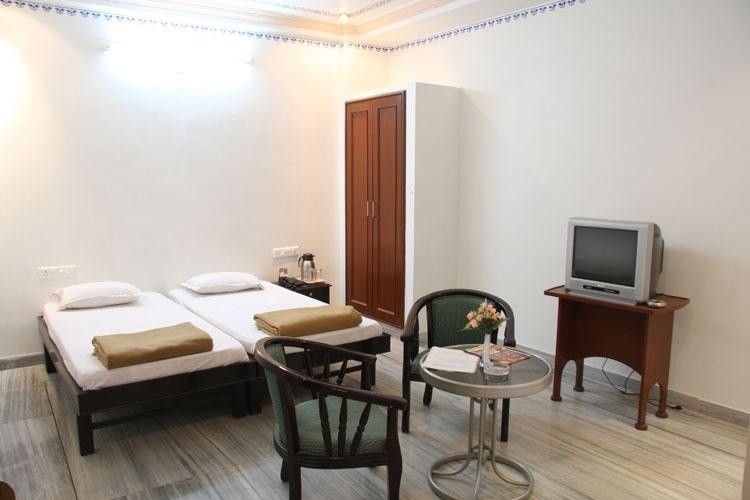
[338,12,350,24]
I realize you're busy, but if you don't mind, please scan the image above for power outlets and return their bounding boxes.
[273,247,299,259]
[39,266,74,280]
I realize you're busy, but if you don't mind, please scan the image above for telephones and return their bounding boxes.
[279,276,304,288]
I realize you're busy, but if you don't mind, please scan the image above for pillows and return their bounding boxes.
[54,281,147,311]
[181,271,262,295]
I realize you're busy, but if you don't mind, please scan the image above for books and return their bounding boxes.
[422,346,480,374]
[465,341,531,366]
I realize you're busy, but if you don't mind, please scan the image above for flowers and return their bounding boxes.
[464,298,510,359]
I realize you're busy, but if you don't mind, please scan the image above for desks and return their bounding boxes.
[419,344,552,500]
[545,284,689,430]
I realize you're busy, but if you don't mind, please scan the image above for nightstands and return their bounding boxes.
[279,279,332,304]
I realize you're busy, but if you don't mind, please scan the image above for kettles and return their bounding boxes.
[297,254,315,281]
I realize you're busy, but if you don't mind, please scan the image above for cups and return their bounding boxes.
[315,269,326,281]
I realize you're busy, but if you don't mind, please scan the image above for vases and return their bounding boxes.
[479,333,495,367]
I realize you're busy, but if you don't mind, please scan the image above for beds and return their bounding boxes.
[38,291,247,454]
[166,280,390,414]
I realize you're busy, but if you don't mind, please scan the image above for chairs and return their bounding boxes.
[400,289,516,442]
[254,335,409,500]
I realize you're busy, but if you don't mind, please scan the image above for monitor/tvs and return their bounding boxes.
[564,218,663,308]
